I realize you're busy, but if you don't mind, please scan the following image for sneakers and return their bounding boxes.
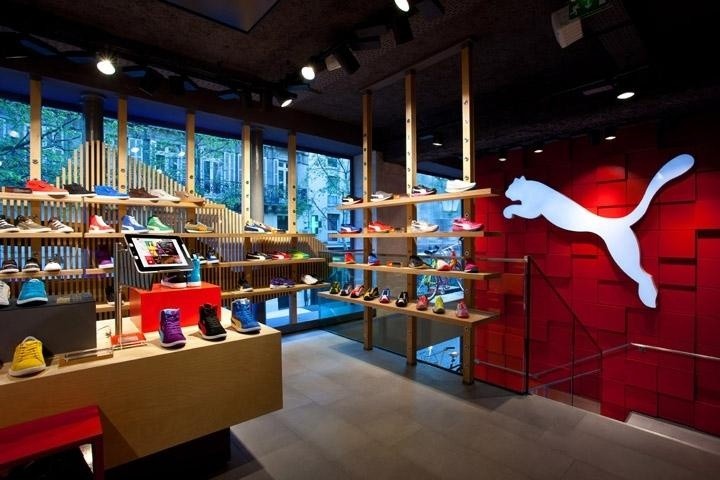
[0,280,11,305]
[244,223,272,234]
[198,304,227,340]
[452,217,484,231]
[26,180,204,203]
[370,190,393,202]
[455,300,469,318]
[344,251,479,273]
[368,221,395,234]
[8,336,46,377]
[330,280,445,314]
[0,253,62,274]
[0,215,74,232]
[342,194,363,205]
[161,259,201,288]
[410,220,440,234]
[300,272,325,285]
[105,285,124,306]
[445,179,477,193]
[230,298,261,333]
[16,278,49,305]
[246,248,312,261]
[236,278,254,291]
[411,185,436,197]
[120,215,174,233]
[340,224,362,234]
[191,249,219,265]
[158,307,186,348]
[184,221,214,233]
[96,244,114,269]
[88,215,116,233]
[270,276,295,289]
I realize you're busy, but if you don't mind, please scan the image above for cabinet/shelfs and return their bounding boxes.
[317,47,500,386]
[0,180,331,479]
